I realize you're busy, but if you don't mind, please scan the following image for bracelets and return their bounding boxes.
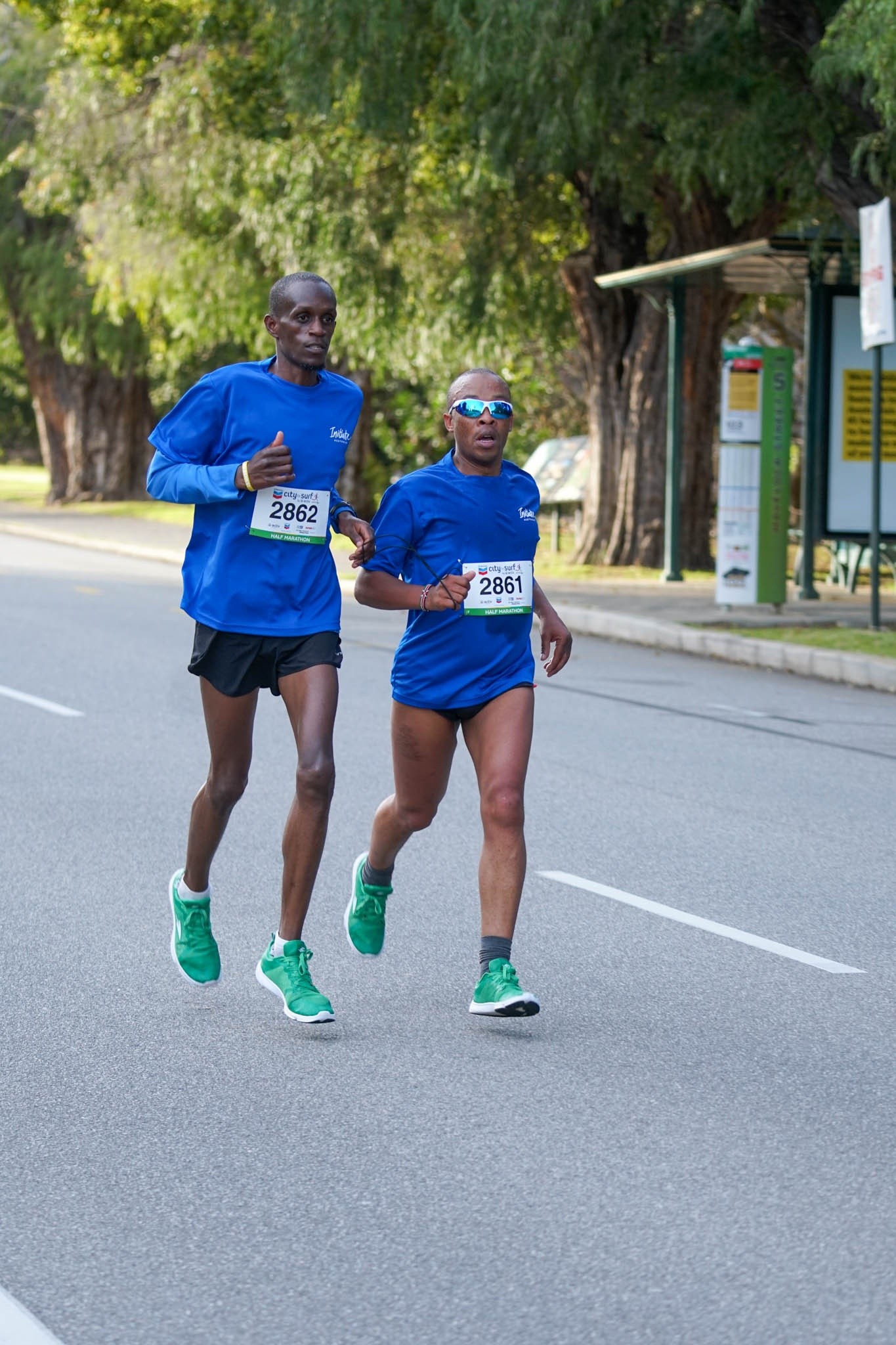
[240,461,259,493]
[420,583,431,612]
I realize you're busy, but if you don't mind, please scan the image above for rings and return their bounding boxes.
[374,545,377,552]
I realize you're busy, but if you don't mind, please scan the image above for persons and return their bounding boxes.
[346,370,573,1015]
[146,272,373,1023]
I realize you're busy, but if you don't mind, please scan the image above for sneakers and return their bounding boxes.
[468,958,540,1017]
[255,932,336,1023]
[343,851,394,959]
[168,867,222,987]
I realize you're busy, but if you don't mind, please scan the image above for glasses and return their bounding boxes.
[448,398,514,420]
